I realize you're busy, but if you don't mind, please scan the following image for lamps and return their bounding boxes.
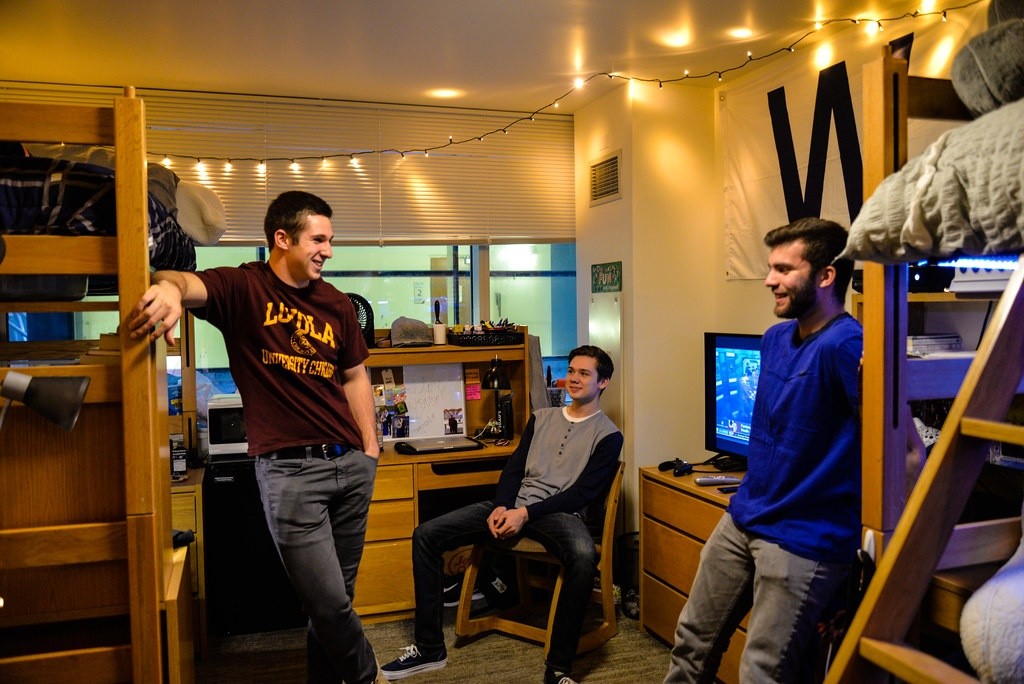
[0,370,91,430]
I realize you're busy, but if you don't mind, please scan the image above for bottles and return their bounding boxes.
[495,390,515,440]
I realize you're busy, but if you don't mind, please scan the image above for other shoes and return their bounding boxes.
[342,649,392,684]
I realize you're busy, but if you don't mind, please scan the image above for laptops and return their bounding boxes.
[404,435,478,452]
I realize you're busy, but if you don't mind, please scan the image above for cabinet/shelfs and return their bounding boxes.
[639,462,751,684]
[352,324,528,624]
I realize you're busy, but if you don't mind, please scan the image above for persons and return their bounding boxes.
[123,189,388,684]
[661,217,862,684]
[380,345,624,683]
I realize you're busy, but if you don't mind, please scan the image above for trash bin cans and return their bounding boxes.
[615,532,639,620]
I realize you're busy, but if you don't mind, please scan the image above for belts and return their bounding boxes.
[259,443,351,461]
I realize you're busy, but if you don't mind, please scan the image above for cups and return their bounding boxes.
[433,324,447,345]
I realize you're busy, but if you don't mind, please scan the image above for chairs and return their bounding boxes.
[455,460,625,660]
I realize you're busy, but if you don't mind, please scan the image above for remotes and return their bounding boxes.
[694,475,742,486]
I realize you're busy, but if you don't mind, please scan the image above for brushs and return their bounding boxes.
[434,300,446,344]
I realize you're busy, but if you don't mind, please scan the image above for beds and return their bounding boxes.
[820,56,1024,684]
[0,87,206,684]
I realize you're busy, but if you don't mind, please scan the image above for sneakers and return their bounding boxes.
[380,644,448,681]
[544,667,579,684]
[443,582,485,607]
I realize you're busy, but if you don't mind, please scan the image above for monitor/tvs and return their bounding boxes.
[541,356,573,405]
[704,332,765,462]
[168,367,241,438]
[6,311,73,343]
